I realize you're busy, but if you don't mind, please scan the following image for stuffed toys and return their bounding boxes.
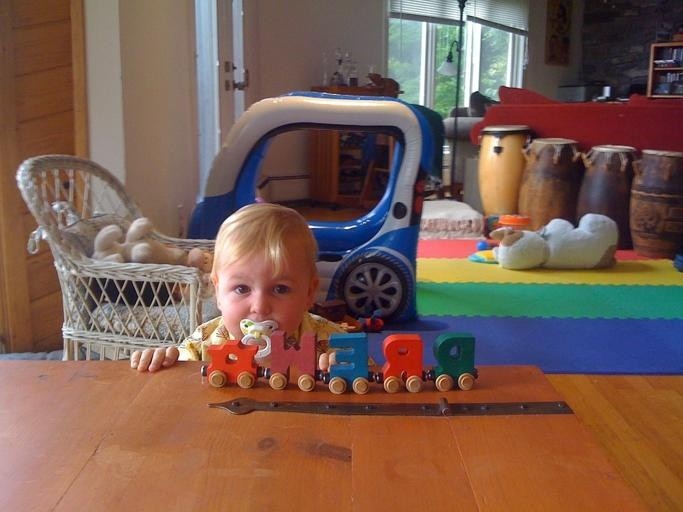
[490,212,618,271]
[92,216,213,274]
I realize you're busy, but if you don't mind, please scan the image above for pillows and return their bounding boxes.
[468,90,498,117]
[629,93,647,103]
[499,84,557,106]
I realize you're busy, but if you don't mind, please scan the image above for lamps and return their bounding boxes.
[436,1,466,199]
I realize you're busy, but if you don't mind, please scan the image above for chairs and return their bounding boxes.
[16,151,216,360]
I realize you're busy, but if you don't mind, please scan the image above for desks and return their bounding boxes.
[1,355,650,512]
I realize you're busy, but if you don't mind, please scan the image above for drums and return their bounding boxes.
[476,124,530,216]
[517,138,584,233]
[628,150,682,258]
[576,145,638,250]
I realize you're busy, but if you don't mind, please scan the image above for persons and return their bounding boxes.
[130,204,348,373]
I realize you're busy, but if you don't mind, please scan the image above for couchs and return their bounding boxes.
[468,103,683,151]
[443,105,485,188]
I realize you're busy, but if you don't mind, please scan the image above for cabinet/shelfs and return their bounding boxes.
[646,42,683,99]
[310,85,386,211]
